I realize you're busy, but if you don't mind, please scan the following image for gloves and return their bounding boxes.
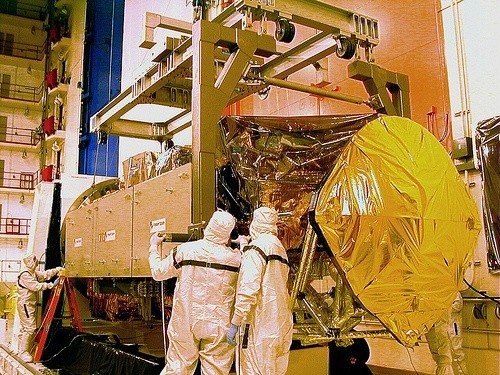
[226,324,238,346]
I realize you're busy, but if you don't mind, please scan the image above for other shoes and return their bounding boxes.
[20,351,33,363]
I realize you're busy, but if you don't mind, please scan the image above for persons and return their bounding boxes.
[446,291,467,375]
[421,295,454,375]
[16,254,66,363]
[225,206,294,375]
[147,211,252,375]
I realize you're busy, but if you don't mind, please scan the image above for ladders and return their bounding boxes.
[32,270,82,362]
